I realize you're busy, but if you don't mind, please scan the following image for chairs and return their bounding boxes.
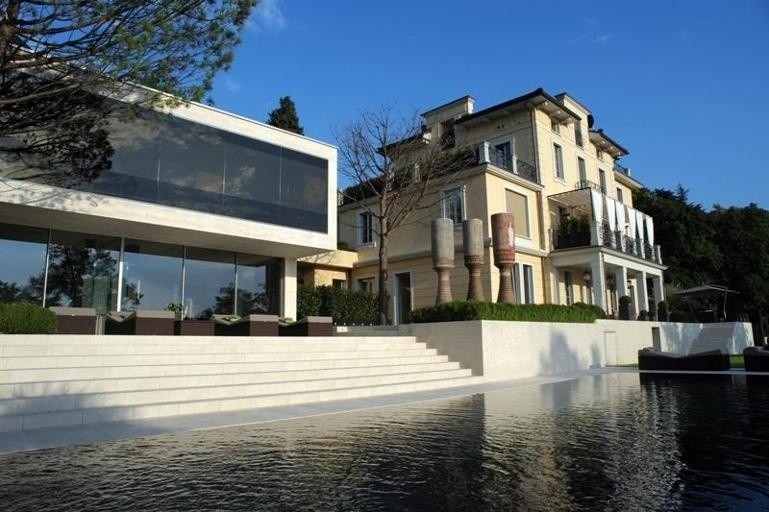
[638,346,730,371]
[743,346,769,372]
[106,311,175,335]
[279,317,333,336]
[49,307,96,334]
[210,314,279,336]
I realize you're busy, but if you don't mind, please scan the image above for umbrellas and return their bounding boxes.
[672,281,741,309]
